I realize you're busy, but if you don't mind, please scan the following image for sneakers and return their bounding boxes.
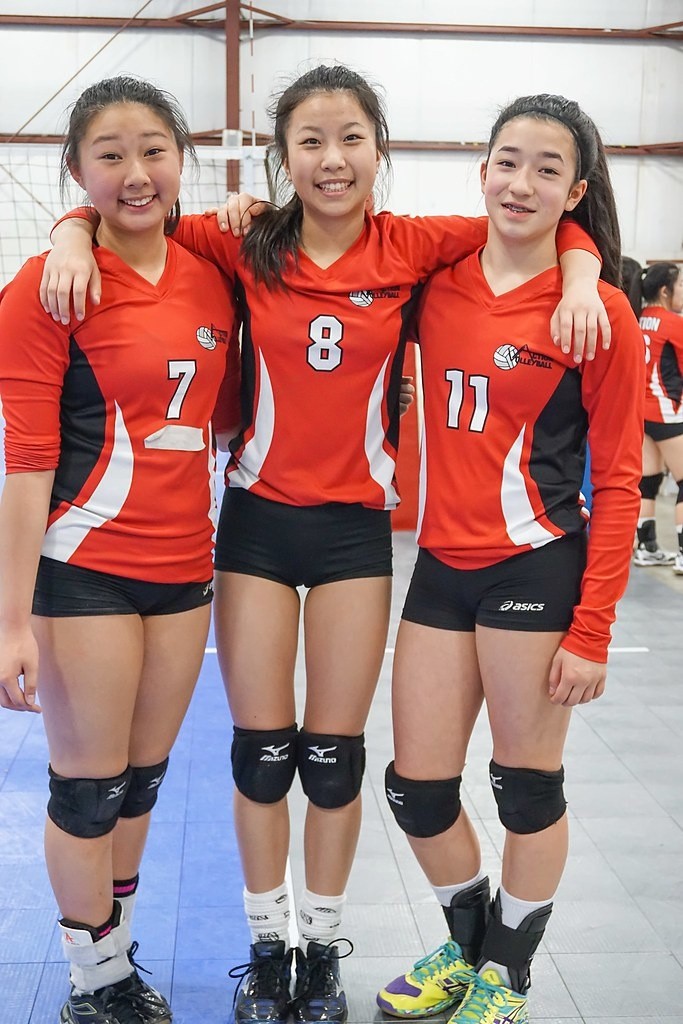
[633,542,677,566]
[446,969,530,1024]
[286,939,354,1024]
[59,941,173,1024]
[672,551,683,574]
[229,940,294,1024]
[376,935,477,1019]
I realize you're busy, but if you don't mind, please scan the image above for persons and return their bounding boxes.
[375,93,647,1024]
[41,64,611,1023]
[618,256,683,575]
[1,77,415,1024]
